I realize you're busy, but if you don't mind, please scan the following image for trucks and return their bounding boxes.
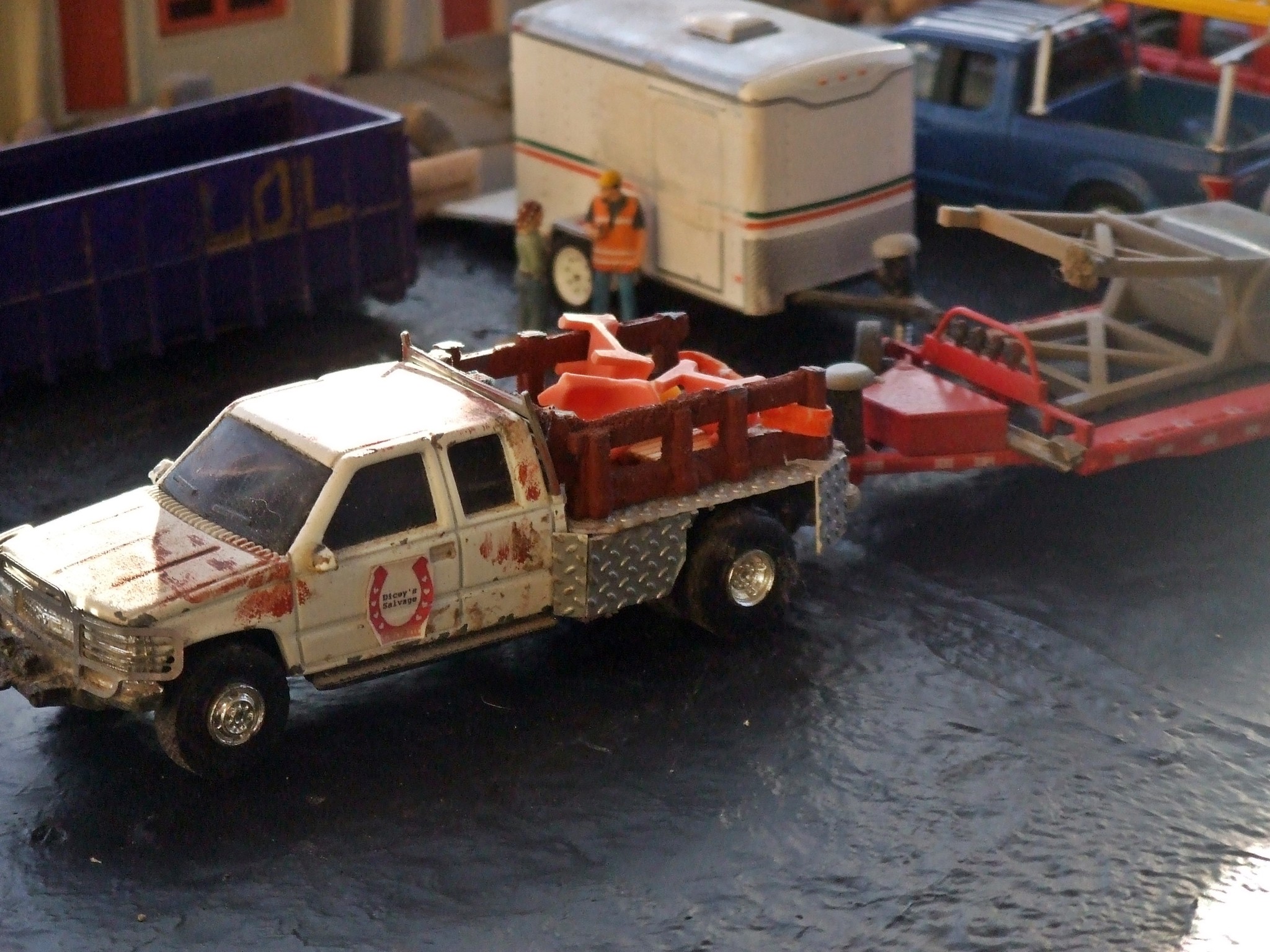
[850,1,1269,241]
[0,312,862,780]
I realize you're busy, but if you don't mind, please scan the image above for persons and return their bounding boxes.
[507,169,648,341]
